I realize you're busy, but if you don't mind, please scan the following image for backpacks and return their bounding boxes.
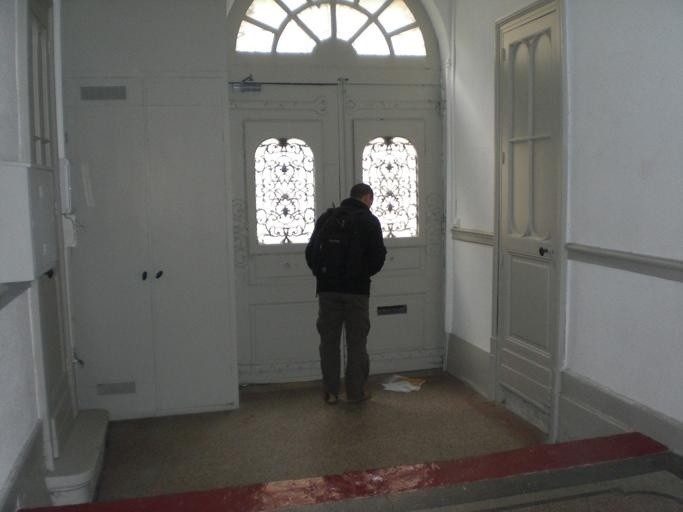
[311,205,370,272]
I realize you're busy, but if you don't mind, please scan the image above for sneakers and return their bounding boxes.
[348,387,371,403]
[323,390,338,403]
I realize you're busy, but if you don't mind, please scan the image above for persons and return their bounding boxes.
[305,184,387,403]
